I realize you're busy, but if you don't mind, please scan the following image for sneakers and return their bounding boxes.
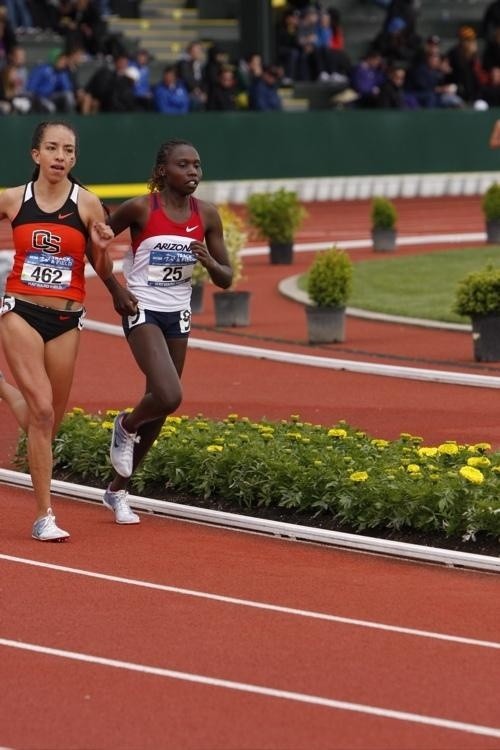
[31,515,71,542]
[110,412,138,478]
[102,481,141,525]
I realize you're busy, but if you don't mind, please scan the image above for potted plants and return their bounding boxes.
[190,199,253,328]
[304,241,355,346]
[369,195,400,252]
[450,256,500,363]
[247,186,310,264]
[480,179,500,245]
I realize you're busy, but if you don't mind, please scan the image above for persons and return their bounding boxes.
[488,119,500,149]
[0,121,114,541]
[86,140,233,524]
